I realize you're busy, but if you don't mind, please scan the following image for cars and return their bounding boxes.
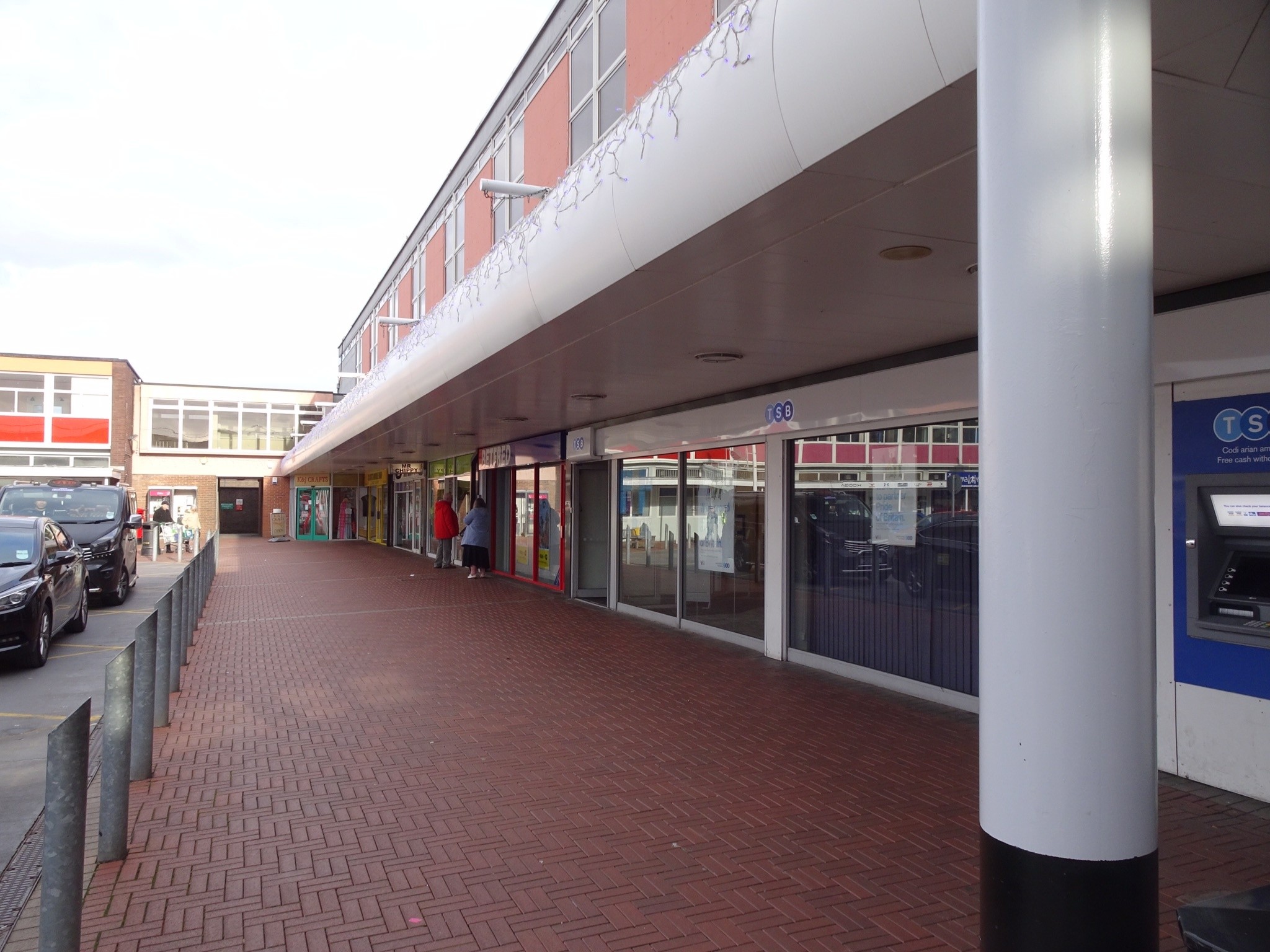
[1,514,90,669]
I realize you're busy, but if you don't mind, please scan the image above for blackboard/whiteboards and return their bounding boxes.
[270,512,286,537]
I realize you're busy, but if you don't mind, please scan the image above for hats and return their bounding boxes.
[162,501,169,505]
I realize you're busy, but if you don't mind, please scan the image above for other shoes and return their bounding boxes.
[443,564,456,568]
[166,550,174,553]
[434,565,442,568]
[467,574,477,579]
[481,574,487,578]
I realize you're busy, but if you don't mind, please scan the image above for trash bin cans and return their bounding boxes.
[141,521,161,556]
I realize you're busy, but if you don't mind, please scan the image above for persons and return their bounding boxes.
[153,501,174,554]
[539,498,560,552]
[703,504,718,541]
[434,492,460,569]
[460,497,492,579]
[182,504,201,552]
[28,497,52,517]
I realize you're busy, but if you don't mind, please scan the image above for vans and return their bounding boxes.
[1,480,142,605]
